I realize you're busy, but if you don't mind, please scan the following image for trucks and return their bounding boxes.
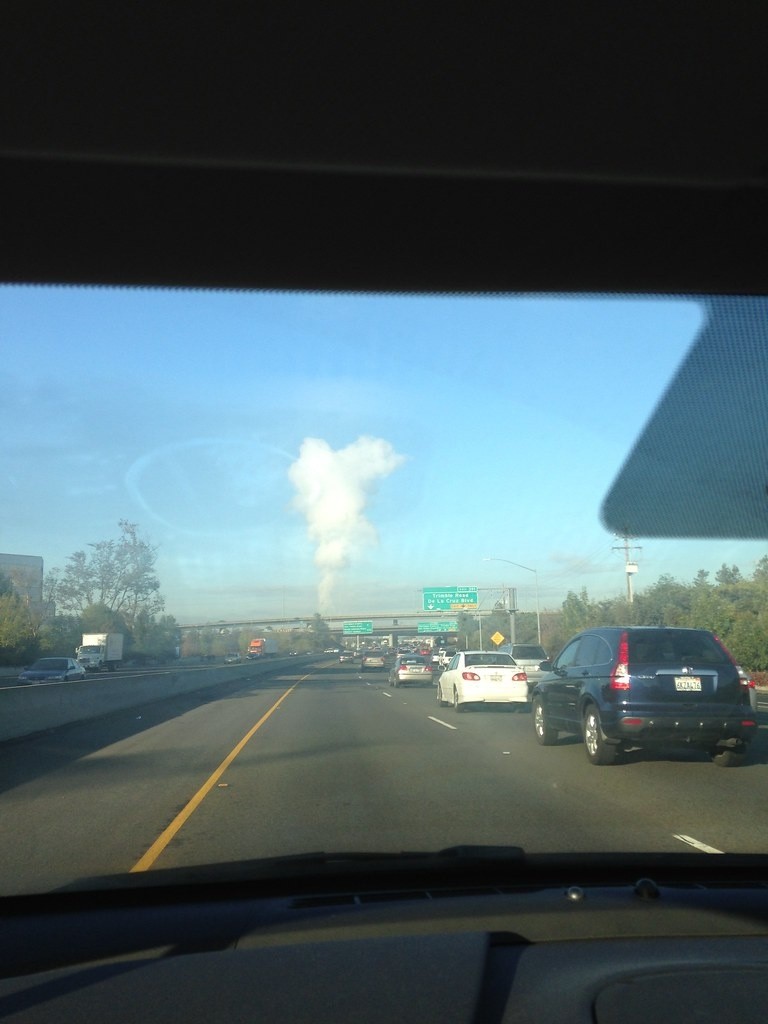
[248,638,280,659]
[74,632,124,672]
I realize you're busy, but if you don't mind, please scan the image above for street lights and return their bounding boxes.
[482,557,541,645]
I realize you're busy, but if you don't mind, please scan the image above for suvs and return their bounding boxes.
[490,641,554,688]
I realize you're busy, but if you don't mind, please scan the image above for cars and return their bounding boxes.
[246,652,260,661]
[16,656,87,686]
[361,650,386,670]
[529,624,757,768]
[287,637,460,667]
[435,650,528,712]
[388,654,433,688]
[224,651,242,664]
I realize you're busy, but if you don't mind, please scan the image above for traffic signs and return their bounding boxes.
[422,586,477,611]
